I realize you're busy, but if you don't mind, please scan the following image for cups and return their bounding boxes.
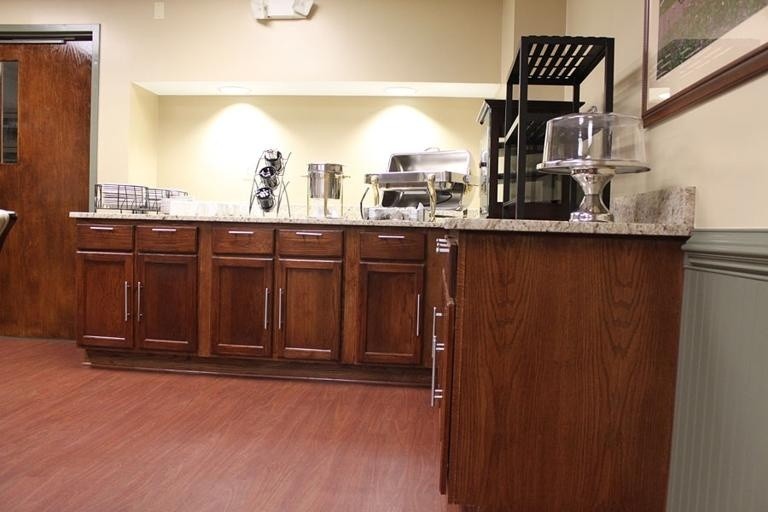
[255,150,281,212]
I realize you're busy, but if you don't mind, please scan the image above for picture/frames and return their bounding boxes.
[641,0,768,128]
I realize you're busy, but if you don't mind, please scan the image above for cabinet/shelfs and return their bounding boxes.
[476,33,615,222]
[350,219,436,387]
[68,213,205,374]
[427,215,696,512]
[205,216,351,377]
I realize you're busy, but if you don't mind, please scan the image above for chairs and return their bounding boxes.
[0,209,17,250]
